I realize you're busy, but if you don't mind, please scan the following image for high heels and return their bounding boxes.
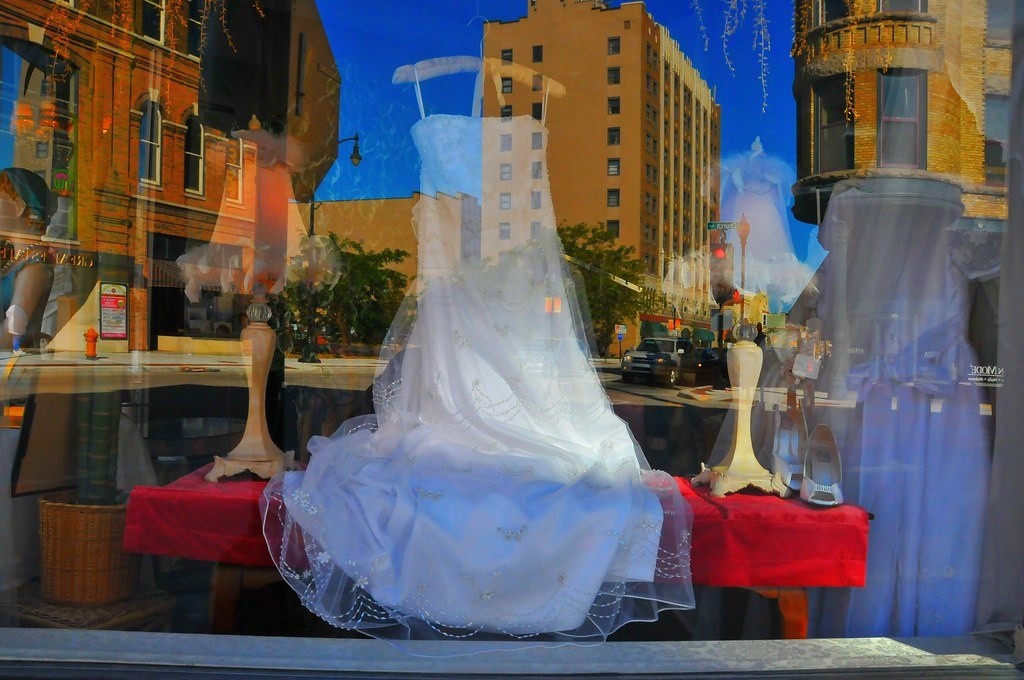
[768,386,810,491]
[799,423,844,506]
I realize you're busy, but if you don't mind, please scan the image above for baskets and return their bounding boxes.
[38,493,140,605]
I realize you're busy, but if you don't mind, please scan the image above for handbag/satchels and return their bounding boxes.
[791,343,821,379]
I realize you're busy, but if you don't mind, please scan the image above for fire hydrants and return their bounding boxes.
[84,328,99,360]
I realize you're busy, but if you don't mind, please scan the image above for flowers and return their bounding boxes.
[284,234,339,322]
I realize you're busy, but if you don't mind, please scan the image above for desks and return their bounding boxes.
[123,461,871,638]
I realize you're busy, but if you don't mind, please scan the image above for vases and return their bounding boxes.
[298,320,321,363]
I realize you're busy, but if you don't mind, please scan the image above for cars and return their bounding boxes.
[623,337,896,395]
[513,337,587,378]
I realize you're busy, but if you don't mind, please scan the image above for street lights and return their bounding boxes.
[299,133,363,363]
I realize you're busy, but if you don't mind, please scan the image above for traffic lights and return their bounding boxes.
[710,244,734,301]
